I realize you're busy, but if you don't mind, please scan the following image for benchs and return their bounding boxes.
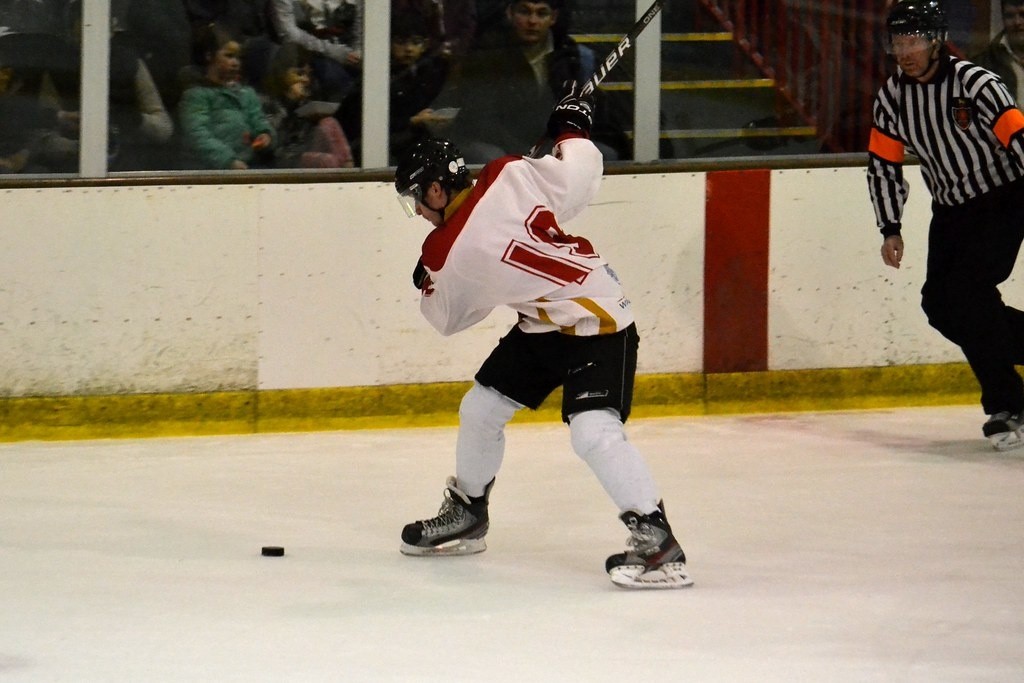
[569,31,816,140]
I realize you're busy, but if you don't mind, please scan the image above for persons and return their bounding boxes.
[0,1,617,173]
[976,0,1024,107]
[864,0,1024,452]
[394,93,696,589]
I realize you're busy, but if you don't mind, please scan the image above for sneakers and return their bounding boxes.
[604,498,694,590]
[399,476,496,556]
[982,410,1024,450]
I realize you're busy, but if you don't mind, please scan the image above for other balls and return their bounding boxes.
[260,545,285,557]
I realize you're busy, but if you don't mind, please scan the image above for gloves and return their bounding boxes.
[413,255,430,290]
[547,79,603,139]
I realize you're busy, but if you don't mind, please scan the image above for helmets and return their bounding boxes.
[394,137,470,212]
[886,0,954,53]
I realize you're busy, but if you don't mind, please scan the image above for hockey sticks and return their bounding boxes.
[523,1,665,161]
